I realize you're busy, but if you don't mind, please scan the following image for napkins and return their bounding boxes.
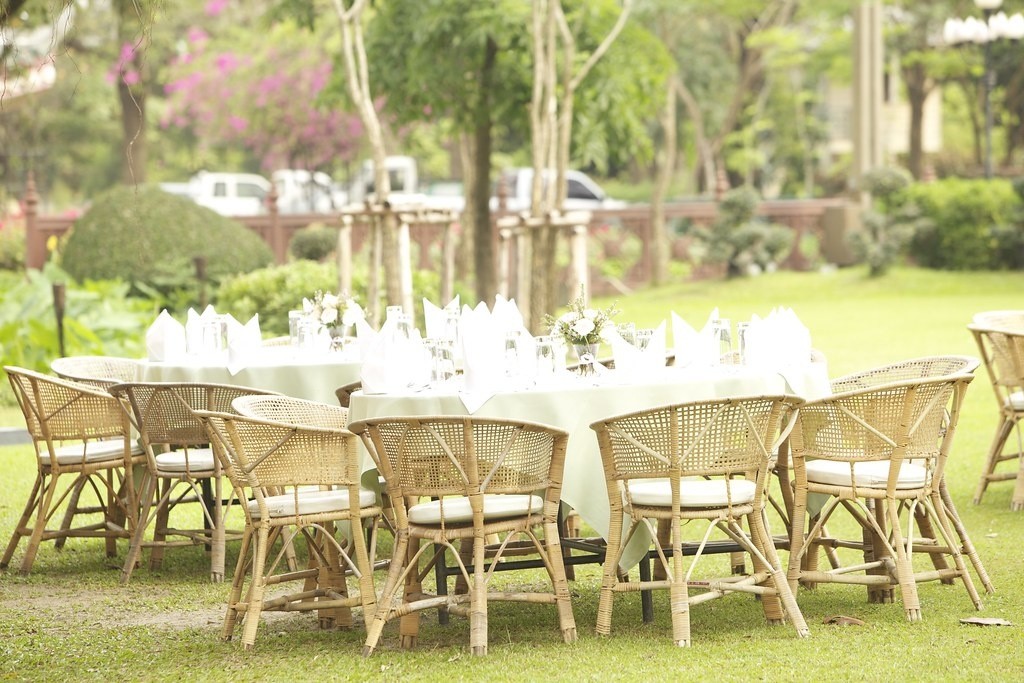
[142,294,811,367]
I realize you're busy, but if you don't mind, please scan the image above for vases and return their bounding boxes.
[568,342,593,362]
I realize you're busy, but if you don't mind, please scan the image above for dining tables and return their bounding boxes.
[348,371,784,636]
[133,346,450,541]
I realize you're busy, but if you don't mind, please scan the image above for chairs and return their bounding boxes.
[968,308,1023,516]
[0,350,995,655]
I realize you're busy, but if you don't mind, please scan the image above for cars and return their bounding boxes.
[158,152,627,218]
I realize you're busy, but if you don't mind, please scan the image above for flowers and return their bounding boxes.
[546,302,608,352]
[291,290,350,343]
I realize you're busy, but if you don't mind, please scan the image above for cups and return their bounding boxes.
[712,319,732,366]
[736,322,752,365]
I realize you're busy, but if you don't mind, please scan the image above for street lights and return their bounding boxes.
[943,0,1024,180]
